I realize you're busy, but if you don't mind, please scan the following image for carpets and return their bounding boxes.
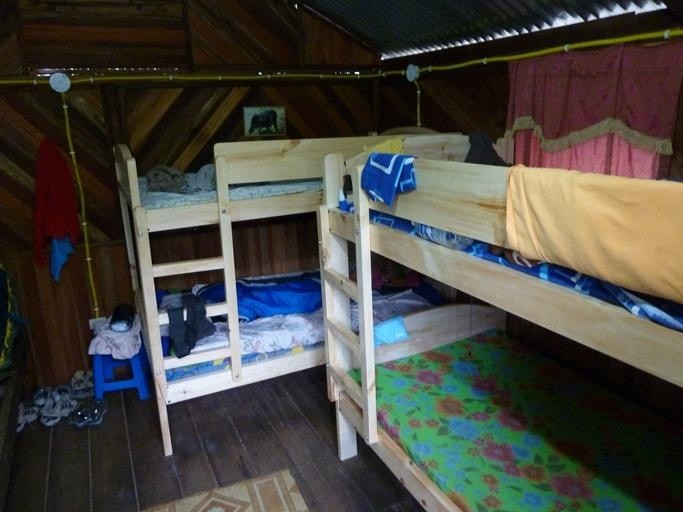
[137,469,310,512]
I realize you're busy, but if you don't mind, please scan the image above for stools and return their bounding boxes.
[94,345,150,400]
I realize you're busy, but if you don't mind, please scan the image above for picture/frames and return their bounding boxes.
[241,106,287,137]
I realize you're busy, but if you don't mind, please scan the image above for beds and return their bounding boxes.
[317,135,683,512]
[111,126,471,456]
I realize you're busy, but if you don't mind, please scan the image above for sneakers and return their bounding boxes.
[17,373,108,431]
